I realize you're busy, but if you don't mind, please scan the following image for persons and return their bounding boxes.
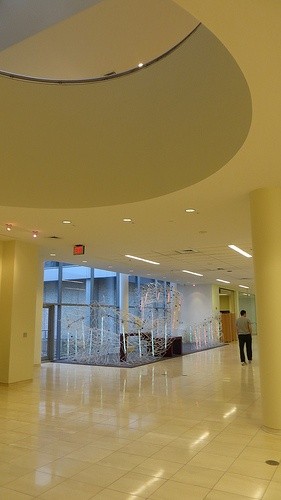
[235,310,253,367]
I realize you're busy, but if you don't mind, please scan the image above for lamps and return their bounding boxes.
[5,224,12,232]
[32,231,38,238]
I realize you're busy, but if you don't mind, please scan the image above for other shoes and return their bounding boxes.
[242,362,247,366]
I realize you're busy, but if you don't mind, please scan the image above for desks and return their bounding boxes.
[155,336,183,358]
